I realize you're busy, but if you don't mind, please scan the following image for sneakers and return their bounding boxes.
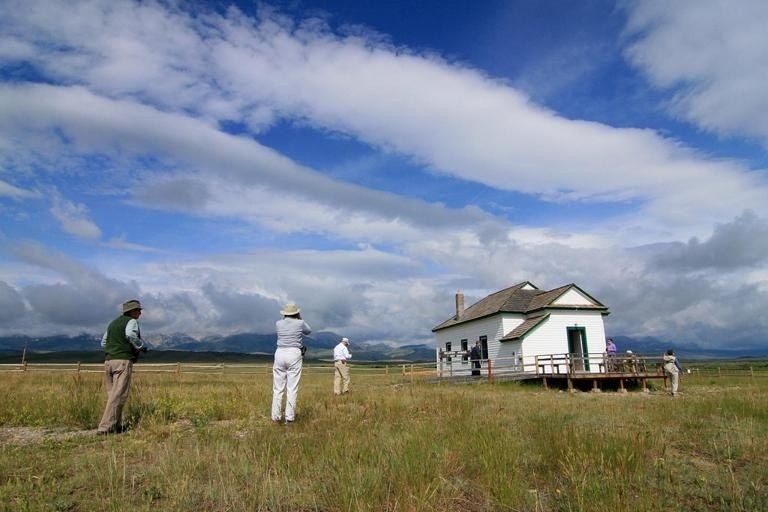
[96,426,126,436]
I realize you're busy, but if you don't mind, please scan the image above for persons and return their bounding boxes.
[332,337,352,397]
[662,349,682,396]
[270,301,312,423]
[605,337,615,372]
[470,339,482,376]
[622,349,636,372]
[95,299,150,436]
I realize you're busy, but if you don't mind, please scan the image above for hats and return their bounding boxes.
[342,337,350,345]
[279,303,300,316]
[475,341,480,344]
[120,300,144,314]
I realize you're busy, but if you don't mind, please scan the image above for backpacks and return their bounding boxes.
[471,347,479,362]
